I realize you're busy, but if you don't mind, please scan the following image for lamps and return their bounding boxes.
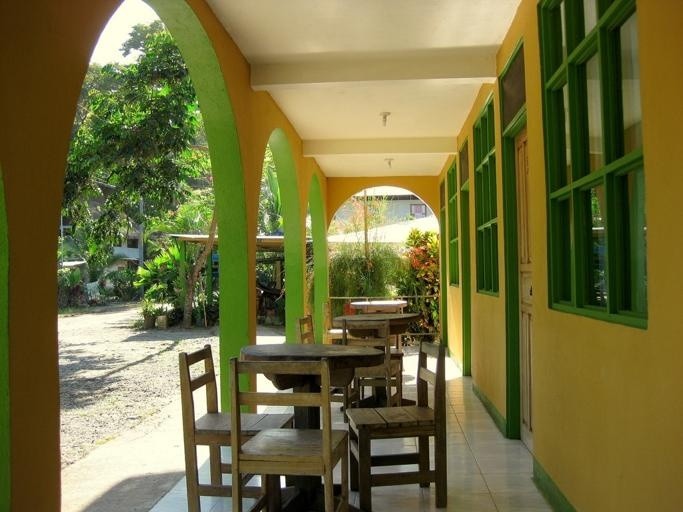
[383,157,395,169]
[379,111,390,125]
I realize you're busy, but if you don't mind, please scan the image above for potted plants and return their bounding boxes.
[139,299,172,331]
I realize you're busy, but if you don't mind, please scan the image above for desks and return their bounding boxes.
[335,312,424,411]
[352,301,409,356]
[242,345,396,512]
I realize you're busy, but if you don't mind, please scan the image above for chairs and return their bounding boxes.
[178,344,295,512]
[296,318,358,415]
[229,357,347,512]
[343,320,402,409]
[365,304,402,373]
[345,337,446,510]
